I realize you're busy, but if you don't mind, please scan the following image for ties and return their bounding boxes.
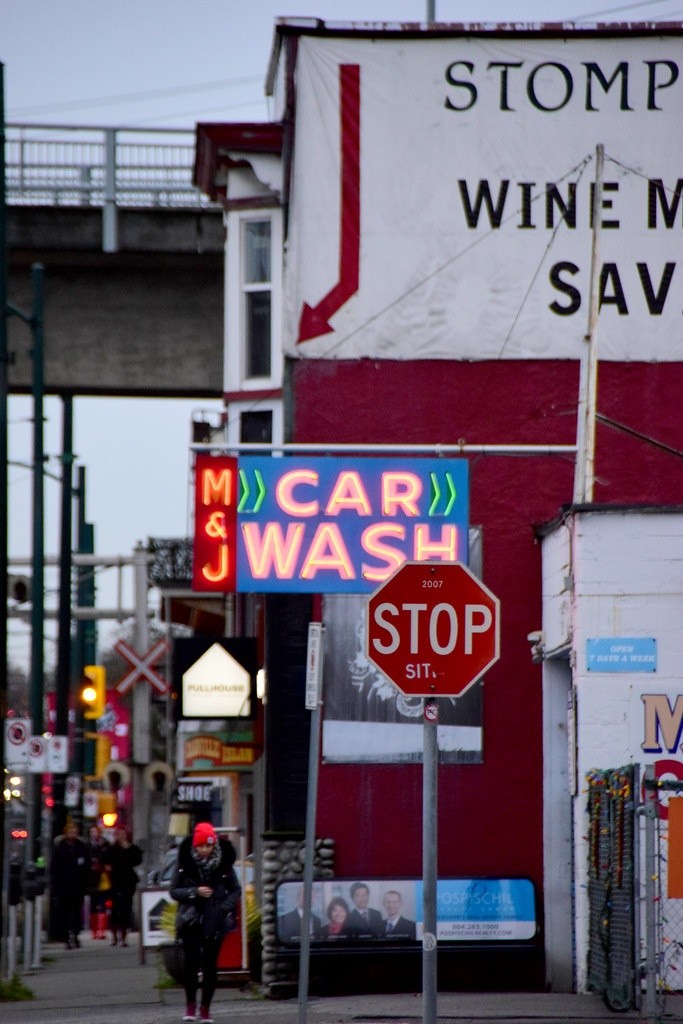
[386,923,393,935]
[361,910,369,924]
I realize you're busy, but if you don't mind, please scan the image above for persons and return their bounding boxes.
[104,826,144,947]
[169,823,242,1023]
[348,883,384,939]
[52,824,89,948]
[383,890,415,939]
[322,897,350,938]
[279,885,321,941]
[85,826,111,939]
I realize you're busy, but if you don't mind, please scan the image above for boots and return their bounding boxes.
[88,913,107,941]
[110,926,129,948]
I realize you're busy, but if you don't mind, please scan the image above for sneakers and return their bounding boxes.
[199,1005,212,1023]
[183,1002,198,1021]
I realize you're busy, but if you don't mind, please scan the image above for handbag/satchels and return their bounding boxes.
[96,873,111,891]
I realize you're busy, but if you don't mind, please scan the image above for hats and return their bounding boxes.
[192,822,217,848]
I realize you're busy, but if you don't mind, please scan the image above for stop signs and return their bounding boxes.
[366,561,500,699]
